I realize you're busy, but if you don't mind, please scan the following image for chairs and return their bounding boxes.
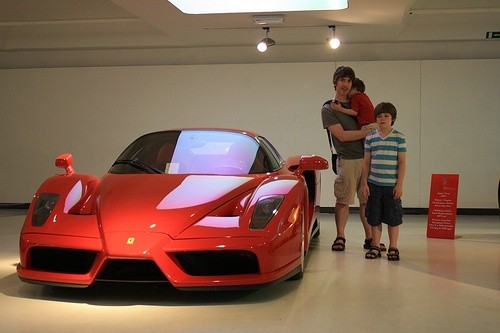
[159,144,192,165]
[227,145,257,172]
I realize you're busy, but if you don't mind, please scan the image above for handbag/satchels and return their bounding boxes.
[332,154,337,174]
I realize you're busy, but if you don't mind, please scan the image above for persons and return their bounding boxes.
[321,65,386,252]
[360,102,407,261]
[331,78,377,131]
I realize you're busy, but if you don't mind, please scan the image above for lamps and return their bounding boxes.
[254,29,276,55]
[326,25,341,50]
[250,14,284,25]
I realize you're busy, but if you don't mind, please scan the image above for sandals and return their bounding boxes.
[364,238,386,250]
[386,247,399,260]
[365,247,382,259]
[332,237,345,251]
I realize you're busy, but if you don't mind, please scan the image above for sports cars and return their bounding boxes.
[16,127,329,295]
